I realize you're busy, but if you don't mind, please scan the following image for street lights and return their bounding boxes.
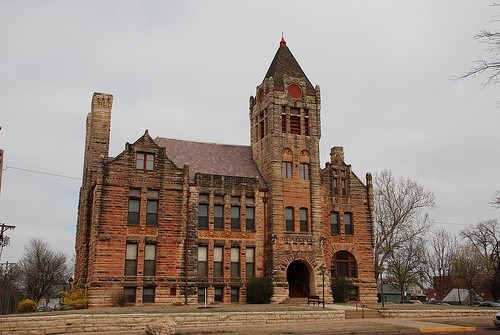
[319,263,328,308]
[378,263,385,307]
[68,277,73,296]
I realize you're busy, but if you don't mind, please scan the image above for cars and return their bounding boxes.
[479,301,500,307]
[492,311,500,329]
[472,301,483,307]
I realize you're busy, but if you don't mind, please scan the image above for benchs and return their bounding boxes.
[307,295,325,308]
